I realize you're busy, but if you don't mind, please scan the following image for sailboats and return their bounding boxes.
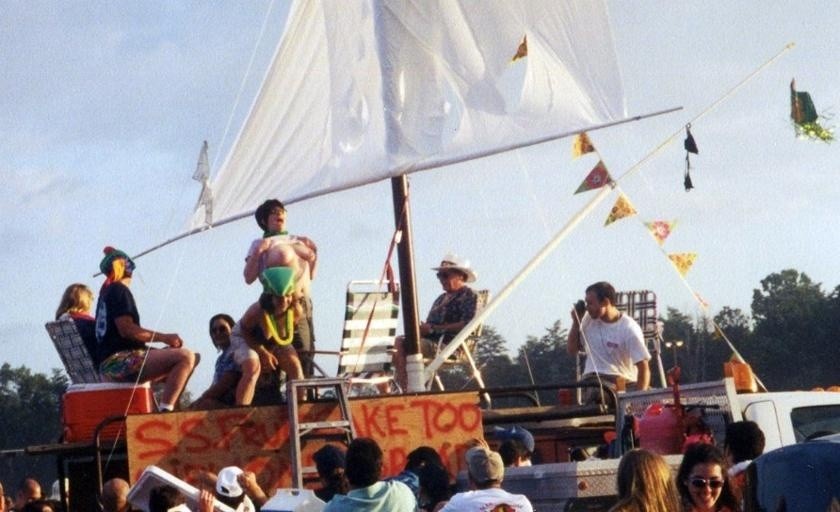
[67,2,683,512]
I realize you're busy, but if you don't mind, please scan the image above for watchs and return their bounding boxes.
[428,324,436,338]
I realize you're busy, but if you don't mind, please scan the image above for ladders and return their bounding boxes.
[286,377,359,494]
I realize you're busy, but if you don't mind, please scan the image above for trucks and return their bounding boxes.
[483,375,839,512]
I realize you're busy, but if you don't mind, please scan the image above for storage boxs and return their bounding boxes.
[60,377,157,444]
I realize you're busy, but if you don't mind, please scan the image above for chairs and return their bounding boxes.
[575,288,671,406]
[44,316,104,383]
[420,290,494,410]
[291,278,404,395]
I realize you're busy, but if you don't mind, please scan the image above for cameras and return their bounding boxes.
[575,298,586,313]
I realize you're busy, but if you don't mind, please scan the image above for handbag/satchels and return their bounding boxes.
[678,432,711,453]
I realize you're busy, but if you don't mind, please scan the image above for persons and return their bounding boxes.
[229,266,308,407]
[413,458,455,512]
[21,500,56,512]
[606,446,683,512]
[167,467,221,512]
[215,465,269,511]
[562,280,662,416]
[181,313,245,408]
[245,199,319,400]
[294,444,349,512]
[93,246,201,414]
[499,436,530,468]
[7,477,43,512]
[55,283,98,373]
[319,437,420,512]
[675,441,743,512]
[382,446,440,500]
[717,420,765,512]
[435,436,534,512]
[102,478,131,511]
[392,252,478,395]
[148,484,186,512]
[494,426,535,466]
[50,473,70,502]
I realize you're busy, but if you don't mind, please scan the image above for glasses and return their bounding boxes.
[270,209,287,214]
[212,326,225,334]
[437,271,450,278]
[687,478,725,487]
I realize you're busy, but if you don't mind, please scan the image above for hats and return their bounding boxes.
[100,246,136,274]
[430,254,477,282]
[492,425,535,453]
[464,446,505,485]
[215,465,244,497]
[311,444,348,476]
[259,267,295,298]
